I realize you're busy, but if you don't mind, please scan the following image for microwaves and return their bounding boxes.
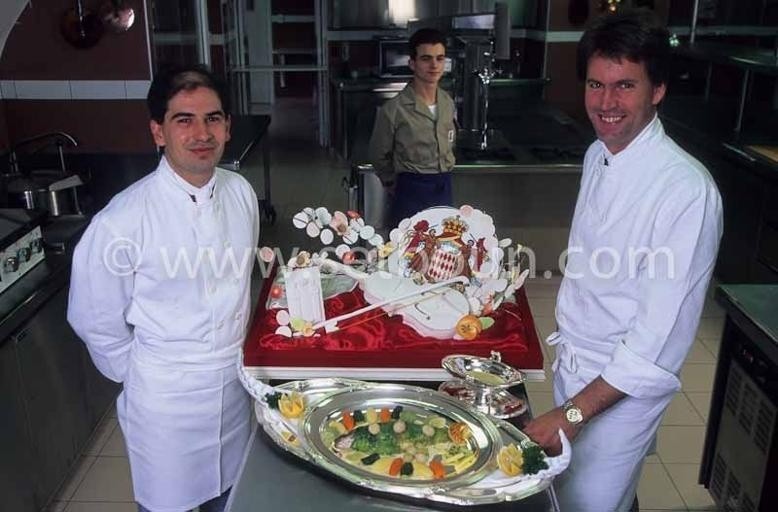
[379,40,456,78]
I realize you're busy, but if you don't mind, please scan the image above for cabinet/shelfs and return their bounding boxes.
[330,81,399,159]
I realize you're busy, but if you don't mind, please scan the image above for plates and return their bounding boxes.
[255,377,556,510]
[299,385,502,490]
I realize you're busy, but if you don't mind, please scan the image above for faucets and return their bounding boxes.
[10,129,78,171]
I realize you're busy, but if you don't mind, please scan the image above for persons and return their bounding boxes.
[65,63,260,511]
[525,15,726,512]
[369,27,458,223]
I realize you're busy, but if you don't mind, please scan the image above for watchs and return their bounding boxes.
[561,398,585,431]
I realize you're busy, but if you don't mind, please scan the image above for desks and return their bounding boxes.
[222,378,561,512]
[216,114,274,226]
